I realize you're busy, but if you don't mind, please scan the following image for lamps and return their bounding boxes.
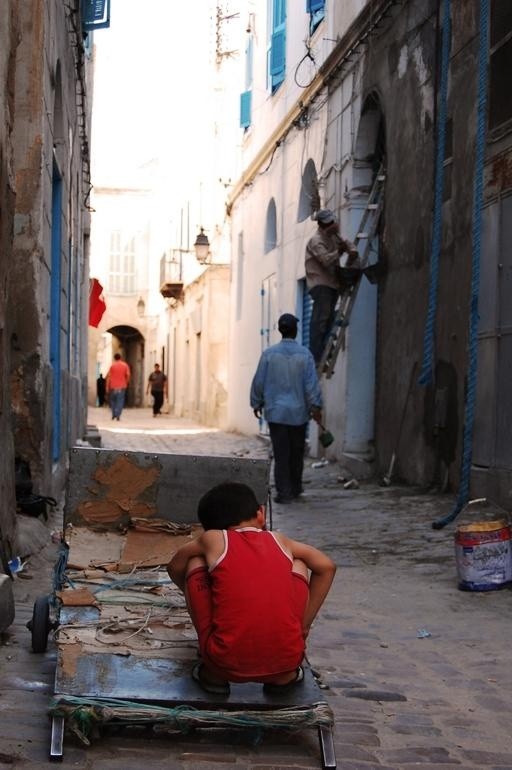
[136,296,160,318]
[193,227,231,266]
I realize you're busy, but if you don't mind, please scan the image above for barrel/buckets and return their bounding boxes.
[453,518,512,592]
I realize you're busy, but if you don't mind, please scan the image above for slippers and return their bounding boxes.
[265,666,303,697]
[189,657,229,695]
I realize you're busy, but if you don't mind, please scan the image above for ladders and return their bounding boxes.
[317,156,386,379]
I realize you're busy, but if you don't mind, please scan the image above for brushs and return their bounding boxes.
[315,422,334,448]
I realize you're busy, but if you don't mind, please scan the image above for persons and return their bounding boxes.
[247,313,324,506]
[145,361,170,419]
[303,208,361,371]
[105,351,132,421]
[164,481,336,697]
[96,374,105,410]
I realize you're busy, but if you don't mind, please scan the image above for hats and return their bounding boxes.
[278,313,299,327]
[314,209,335,224]
